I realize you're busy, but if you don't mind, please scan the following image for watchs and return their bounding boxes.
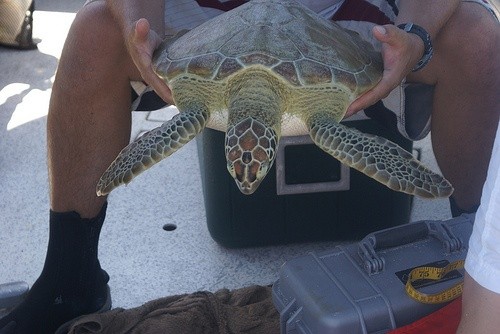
[394,21,433,74]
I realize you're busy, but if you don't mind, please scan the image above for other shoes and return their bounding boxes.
[0,200,112,334]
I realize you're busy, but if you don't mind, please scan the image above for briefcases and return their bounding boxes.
[269,210,476,334]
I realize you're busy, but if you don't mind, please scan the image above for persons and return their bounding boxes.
[454,122,499,334]
[0,0,499,334]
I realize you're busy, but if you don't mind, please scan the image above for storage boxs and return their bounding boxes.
[193,84,416,251]
[272,215,476,334]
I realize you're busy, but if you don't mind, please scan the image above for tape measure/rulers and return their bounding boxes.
[405,259,465,305]
